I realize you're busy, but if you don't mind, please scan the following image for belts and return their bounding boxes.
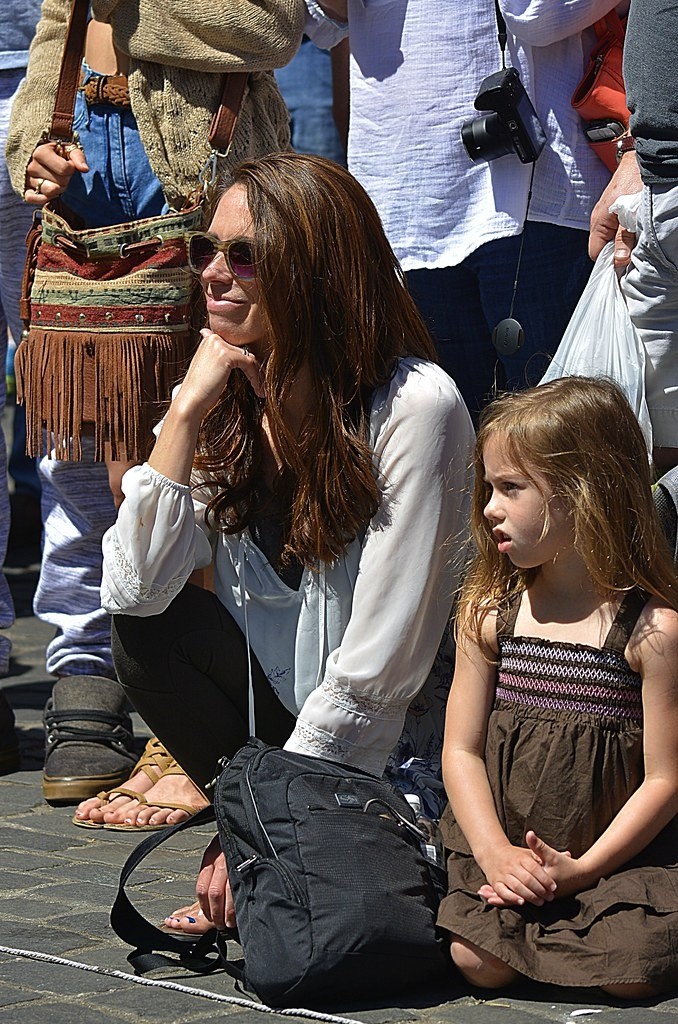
[74,68,130,109]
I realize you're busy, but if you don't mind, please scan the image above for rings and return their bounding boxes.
[34,179,45,192]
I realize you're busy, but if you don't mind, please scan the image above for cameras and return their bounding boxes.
[460,68,548,165]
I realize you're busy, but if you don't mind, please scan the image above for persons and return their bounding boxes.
[441,375,677,1000]
[98,151,476,934]
[0,1,678,831]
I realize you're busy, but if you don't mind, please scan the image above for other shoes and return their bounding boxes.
[156,898,217,936]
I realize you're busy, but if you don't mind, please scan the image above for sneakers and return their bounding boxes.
[41,673,141,801]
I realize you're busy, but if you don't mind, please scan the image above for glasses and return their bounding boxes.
[185,230,268,280]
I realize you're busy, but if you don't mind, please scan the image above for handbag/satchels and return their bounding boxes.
[570,7,637,175]
[13,181,209,464]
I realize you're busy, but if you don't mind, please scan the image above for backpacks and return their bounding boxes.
[109,736,453,1009]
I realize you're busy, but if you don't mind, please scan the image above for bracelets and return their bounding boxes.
[616,137,638,164]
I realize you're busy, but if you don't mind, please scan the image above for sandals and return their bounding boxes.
[71,734,210,832]
